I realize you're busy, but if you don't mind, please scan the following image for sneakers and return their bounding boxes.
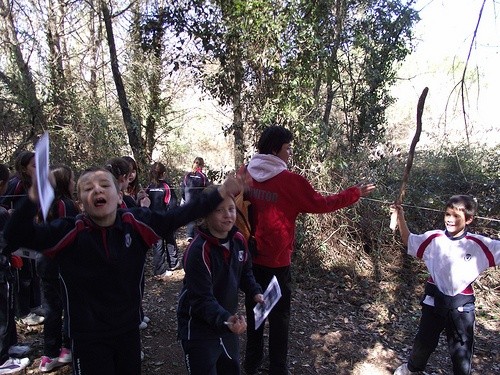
[0,357,30,375]
[60,348,72,363]
[39,356,66,373]
[8,346,31,358]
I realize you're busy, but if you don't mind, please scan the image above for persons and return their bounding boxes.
[389,195,500,375]
[176,185,264,375]
[103,156,213,330]
[232,125,376,375]
[3,159,253,375]
[0,150,81,372]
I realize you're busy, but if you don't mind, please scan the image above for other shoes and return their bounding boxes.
[139,322,148,329]
[143,316,150,323]
[152,271,173,282]
[170,260,180,270]
[14,312,45,326]
[394,363,414,375]
